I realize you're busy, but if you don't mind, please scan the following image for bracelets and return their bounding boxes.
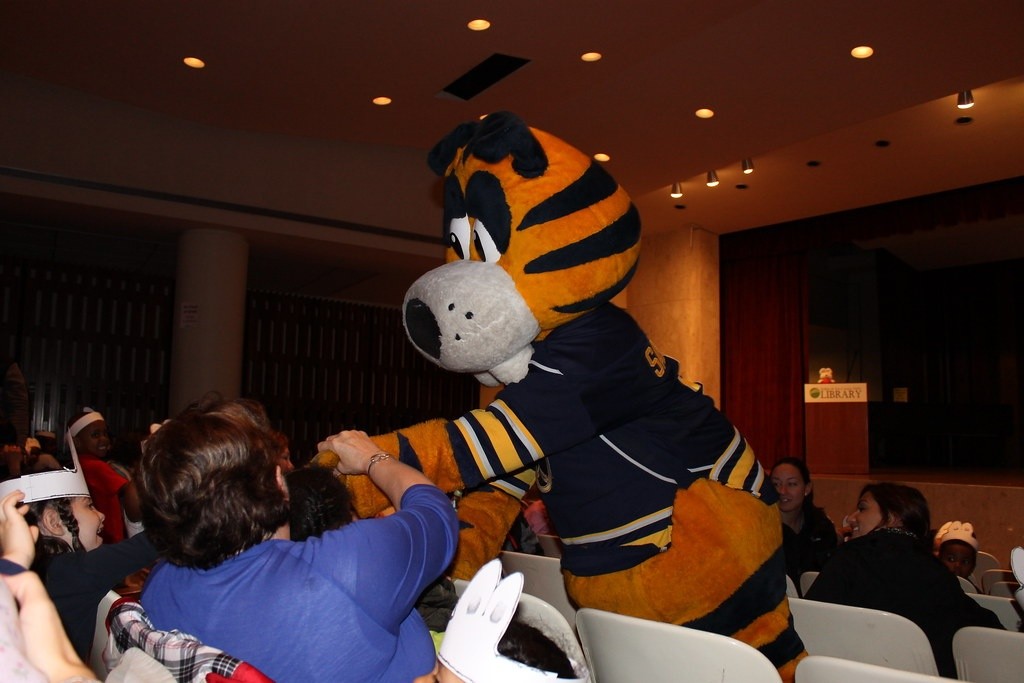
[367,453,391,476]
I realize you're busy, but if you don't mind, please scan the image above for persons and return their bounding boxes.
[138,398,459,683]
[941,521,983,594]
[0,352,29,446]
[0,407,160,683]
[770,456,839,573]
[804,482,1006,680]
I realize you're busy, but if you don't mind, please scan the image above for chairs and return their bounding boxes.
[990,581,1020,598]
[968,550,1001,594]
[788,595,939,677]
[957,576,977,593]
[982,569,1017,596]
[952,626,1024,683]
[575,608,784,683]
[499,549,577,635]
[452,578,589,679]
[537,533,561,558]
[794,655,974,683]
[966,592,1024,633]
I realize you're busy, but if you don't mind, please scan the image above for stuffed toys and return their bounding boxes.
[311,112,808,683]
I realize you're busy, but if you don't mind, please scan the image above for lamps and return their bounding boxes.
[957,90,974,110]
[705,170,720,188]
[741,158,754,174]
[669,182,683,199]
